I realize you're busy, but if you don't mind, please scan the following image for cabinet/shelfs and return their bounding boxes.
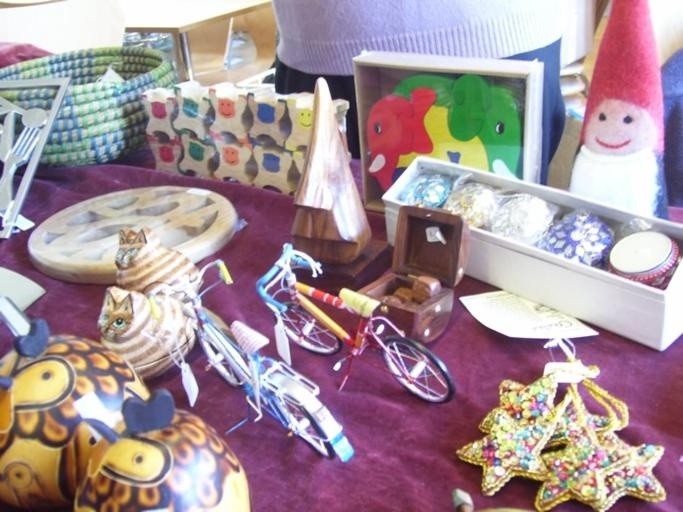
[126,0,276,87]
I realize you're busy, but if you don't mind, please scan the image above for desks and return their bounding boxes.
[0,159,683,512]
